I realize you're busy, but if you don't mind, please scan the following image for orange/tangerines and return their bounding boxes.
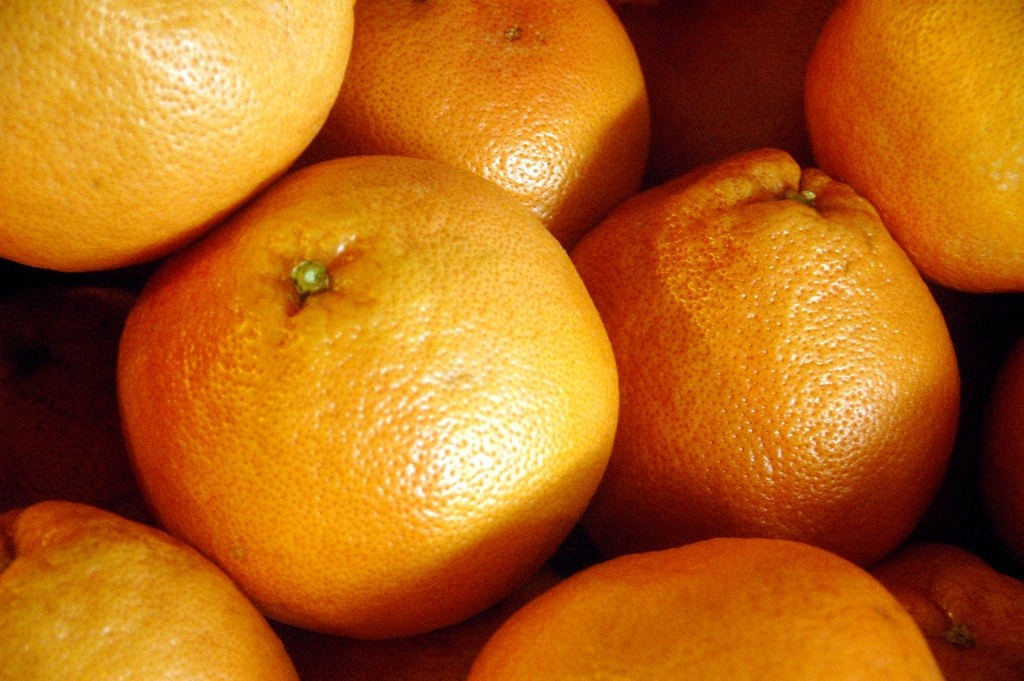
[0,0,1022,681]
